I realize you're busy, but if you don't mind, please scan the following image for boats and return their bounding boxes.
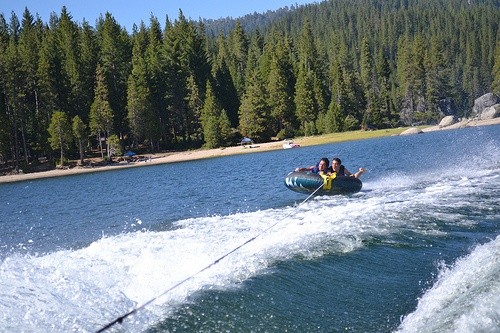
[284,171,362,196]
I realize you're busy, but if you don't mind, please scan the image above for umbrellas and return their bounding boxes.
[242,137,251,148]
[123,150,135,161]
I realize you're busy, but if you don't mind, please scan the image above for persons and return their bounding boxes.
[328,157,365,181]
[298,157,328,180]
